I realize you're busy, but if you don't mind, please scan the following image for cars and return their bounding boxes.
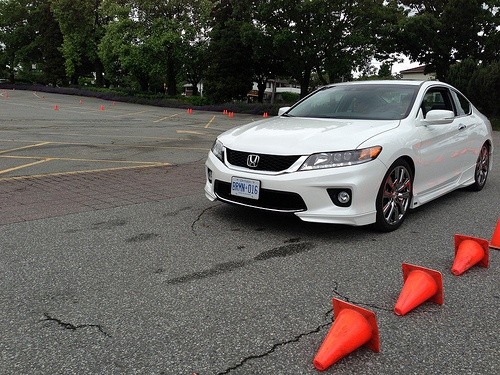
[204,77,494,234]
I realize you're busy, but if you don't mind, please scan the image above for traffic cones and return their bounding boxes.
[223,108,235,118]
[53,104,59,110]
[394,262,443,316]
[186,108,193,113]
[262,112,269,118]
[313,297,381,372]
[450,220,500,276]
[99,105,105,110]
